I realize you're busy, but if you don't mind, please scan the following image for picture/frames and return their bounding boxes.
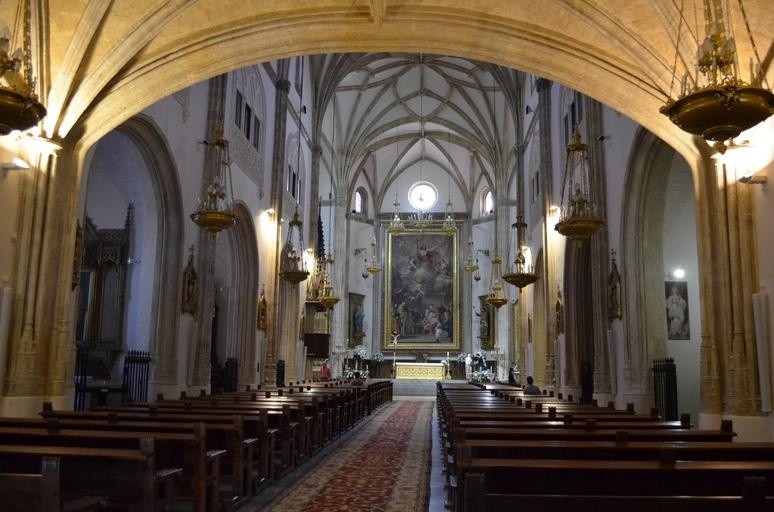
[382,224,462,351]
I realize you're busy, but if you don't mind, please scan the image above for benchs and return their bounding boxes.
[433,378,774,512]
[1,376,393,512]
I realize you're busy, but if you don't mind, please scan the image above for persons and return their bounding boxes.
[351,372,364,386]
[522,376,541,393]
[391,244,453,342]
[508,367,519,384]
[320,362,331,379]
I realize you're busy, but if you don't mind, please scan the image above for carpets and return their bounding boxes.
[258,399,436,511]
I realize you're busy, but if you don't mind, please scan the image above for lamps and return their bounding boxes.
[0,0,46,134]
[278,53,343,308]
[555,84,605,246]
[660,0,773,155]
[192,74,238,239]
[388,52,463,236]
[484,60,538,310]
